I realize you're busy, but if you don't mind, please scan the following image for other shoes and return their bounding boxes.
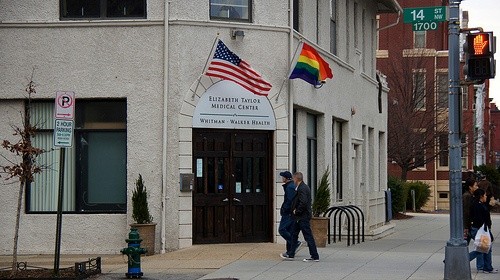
[294,241,306,254]
[303,258,319,262]
[479,269,498,273]
[279,253,294,260]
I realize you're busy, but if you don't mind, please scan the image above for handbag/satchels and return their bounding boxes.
[474,224,491,254]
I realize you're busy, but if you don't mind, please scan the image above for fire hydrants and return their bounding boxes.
[120,227,148,280]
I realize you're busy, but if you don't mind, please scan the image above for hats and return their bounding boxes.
[280,171,292,180]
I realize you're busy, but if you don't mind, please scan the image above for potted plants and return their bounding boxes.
[310,164,332,248]
[129,173,157,256]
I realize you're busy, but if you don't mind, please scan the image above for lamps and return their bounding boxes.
[230,29,244,41]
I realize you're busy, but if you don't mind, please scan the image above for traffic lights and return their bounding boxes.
[465,31,493,80]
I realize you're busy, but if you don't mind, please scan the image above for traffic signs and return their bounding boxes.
[54,119,74,148]
[55,91,75,121]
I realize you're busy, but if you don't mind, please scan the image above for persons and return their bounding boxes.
[443,178,484,271]
[278,171,302,254]
[280,172,320,262]
[468,188,499,274]
[477,174,499,225]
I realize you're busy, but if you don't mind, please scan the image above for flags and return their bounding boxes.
[288,42,333,86]
[204,38,273,97]
